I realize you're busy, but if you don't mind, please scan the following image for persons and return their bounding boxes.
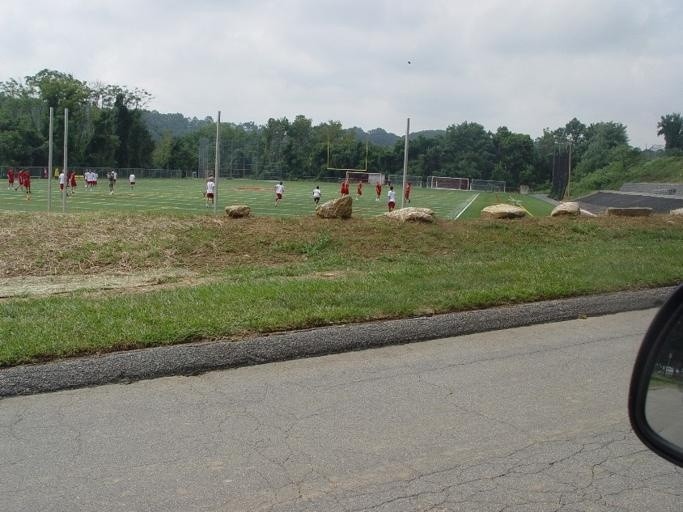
[312,186,320,209]
[340,180,345,197]
[344,180,349,195]
[354,181,361,200]
[404,183,411,203]
[387,186,395,212]
[7,166,136,194]
[206,177,214,208]
[207,174,215,184]
[374,181,381,201]
[273,181,283,206]
